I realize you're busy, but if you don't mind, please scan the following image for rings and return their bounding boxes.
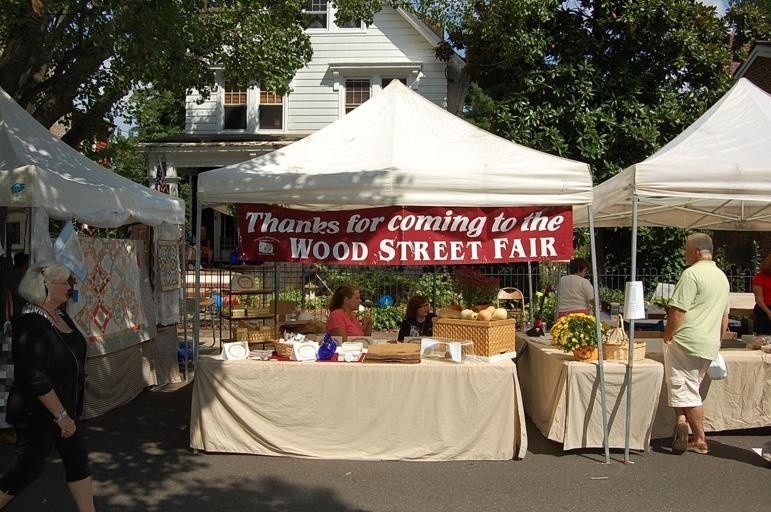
[62,430,65,432]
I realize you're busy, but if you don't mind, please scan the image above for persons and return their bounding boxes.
[397,296,438,342]
[325,285,374,342]
[555,257,595,319]
[0,260,96,512]
[10,252,29,319]
[753,254,771,336]
[662,232,730,454]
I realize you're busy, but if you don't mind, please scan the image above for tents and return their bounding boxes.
[0,82,186,428]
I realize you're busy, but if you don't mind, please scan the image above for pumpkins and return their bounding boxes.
[573,345,599,362]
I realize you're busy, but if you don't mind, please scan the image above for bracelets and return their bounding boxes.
[53,409,68,422]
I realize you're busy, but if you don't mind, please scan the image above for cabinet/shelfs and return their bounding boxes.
[218,260,277,354]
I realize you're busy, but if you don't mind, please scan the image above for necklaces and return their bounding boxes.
[32,304,59,324]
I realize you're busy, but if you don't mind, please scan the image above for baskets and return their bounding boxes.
[273,339,293,357]
[602,314,646,361]
[724,326,738,339]
[232,330,275,342]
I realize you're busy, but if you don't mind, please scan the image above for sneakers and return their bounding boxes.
[672,422,708,454]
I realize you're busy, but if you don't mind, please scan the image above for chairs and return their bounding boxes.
[496,286,527,334]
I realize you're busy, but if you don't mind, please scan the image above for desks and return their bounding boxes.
[512,328,666,454]
[187,296,217,348]
[189,348,531,463]
[645,347,771,442]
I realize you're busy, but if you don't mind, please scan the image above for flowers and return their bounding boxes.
[549,312,607,353]
